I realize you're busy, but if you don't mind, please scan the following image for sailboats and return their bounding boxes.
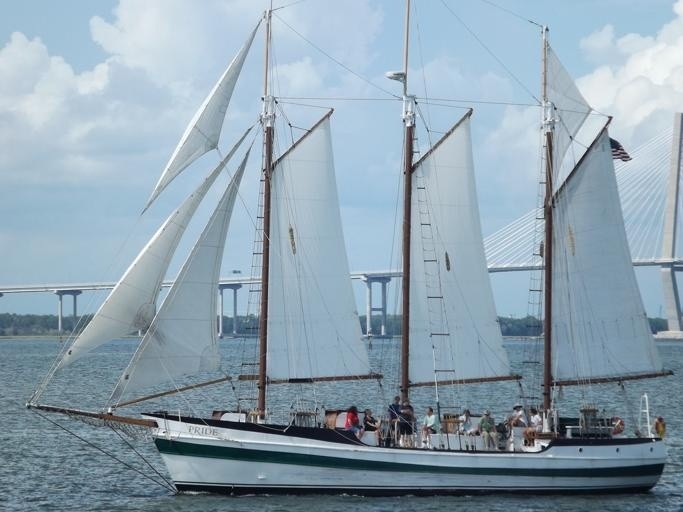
[24,2,683,495]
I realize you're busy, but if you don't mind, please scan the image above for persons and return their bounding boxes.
[478,409,499,452]
[654,416,664,438]
[344,406,364,439]
[421,407,439,449]
[522,407,542,446]
[507,403,529,425]
[361,408,383,446]
[399,399,416,435]
[459,407,476,450]
[386,395,401,447]
